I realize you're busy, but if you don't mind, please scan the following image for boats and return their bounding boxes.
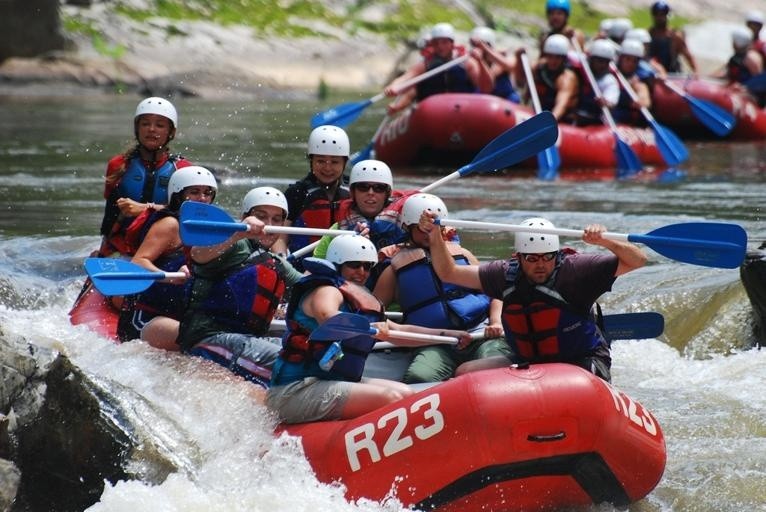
[65,251,670,508]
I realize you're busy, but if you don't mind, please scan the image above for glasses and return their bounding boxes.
[346,262,372,272]
[523,252,557,263]
[357,182,387,195]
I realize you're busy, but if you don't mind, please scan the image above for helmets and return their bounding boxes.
[730,28,754,47]
[544,33,570,56]
[240,186,289,220]
[326,234,379,268]
[601,17,652,57]
[746,8,764,27]
[545,0,570,12]
[589,40,616,61]
[306,124,349,159]
[400,192,448,230]
[432,23,454,43]
[470,26,496,46]
[167,166,218,206]
[133,96,178,128]
[513,216,560,254]
[349,160,392,193]
[651,2,670,15]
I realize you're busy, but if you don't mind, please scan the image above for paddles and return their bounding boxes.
[179,201,361,247]
[521,53,562,168]
[84,258,187,296]
[469,312,663,340]
[306,312,460,346]
[287,110,558,261]
[572,36,643,171]
[434,218,747,269]
[639,61,737,135]
[310,53,471,128]
[609,61,690,166]
[350,95,399,165]
